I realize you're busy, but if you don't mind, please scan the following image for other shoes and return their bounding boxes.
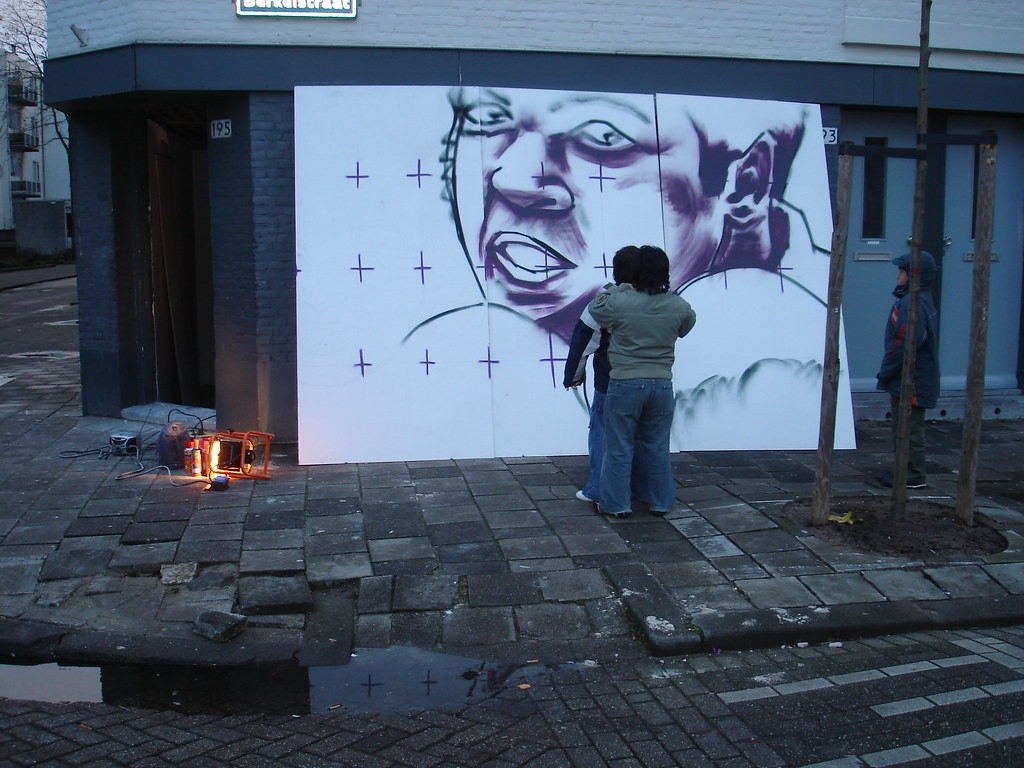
[593,501,631,519]
[877,467,926,489]
[653,511,666,516]
[576,490,594,503]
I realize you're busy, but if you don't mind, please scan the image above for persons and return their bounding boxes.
[563,246,638,502]
[877,251,940,488]
[587,245,696,518]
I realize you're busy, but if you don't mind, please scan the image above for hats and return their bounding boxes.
[892,255,908,267]
